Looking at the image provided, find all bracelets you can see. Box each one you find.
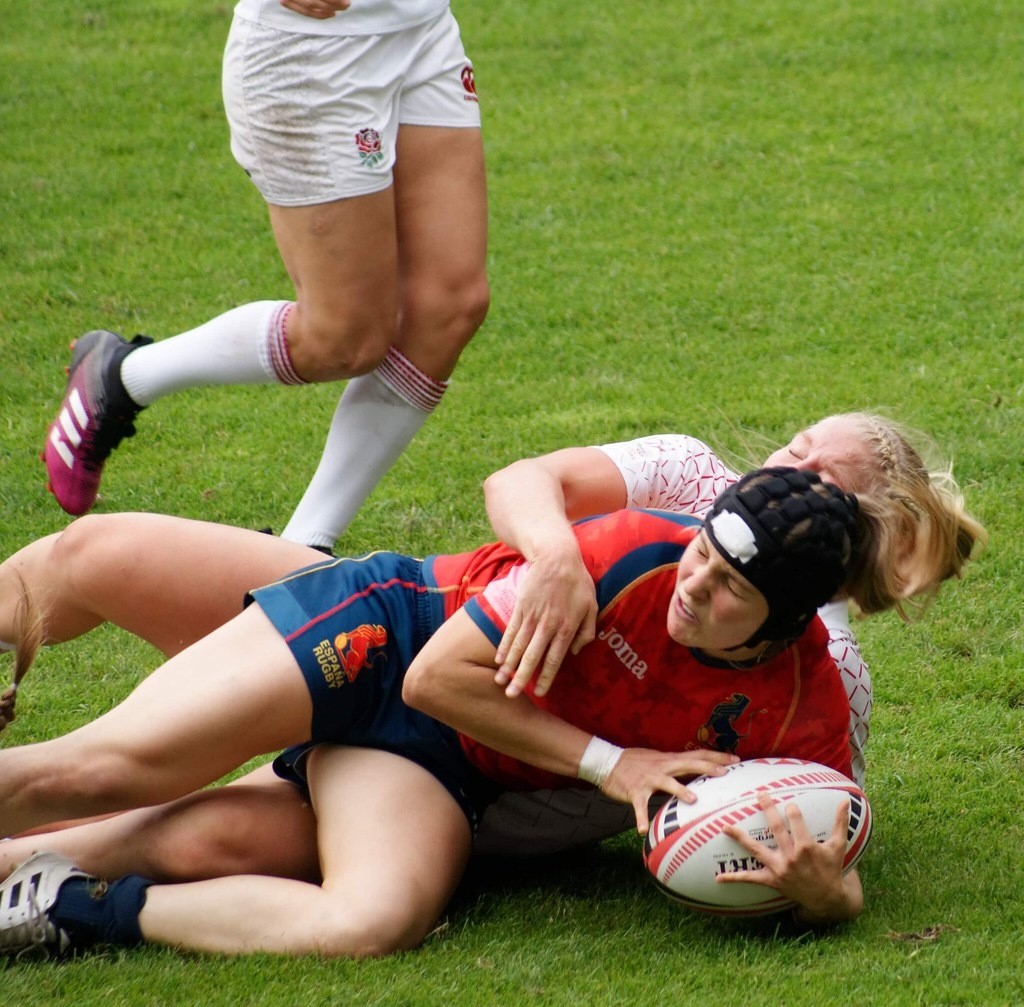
[572,734,626,788]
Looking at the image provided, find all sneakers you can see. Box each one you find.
[41,329,143,517]
[0,846,103,961]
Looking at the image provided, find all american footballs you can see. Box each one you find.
[641,756,873,915]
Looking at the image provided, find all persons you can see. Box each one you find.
[0,410,989,957]
[39,0,490,556]
